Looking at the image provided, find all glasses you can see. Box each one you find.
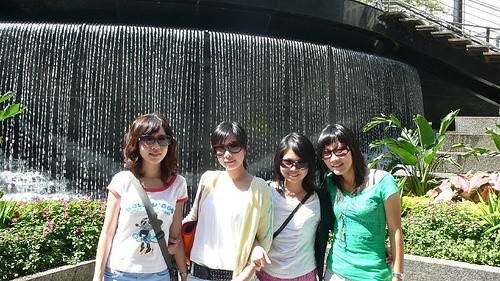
[279,158,309,170]
[138,134,172,145]
[319,145,351,160]
[213,142,243,156]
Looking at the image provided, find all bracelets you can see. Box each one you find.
[179,272,188,277]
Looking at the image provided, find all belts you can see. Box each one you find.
[189,261,233,281]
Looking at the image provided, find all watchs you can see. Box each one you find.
[392,272,405,281]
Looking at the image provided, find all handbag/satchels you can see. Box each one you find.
[181,221,198,266]
[170,254,179,281]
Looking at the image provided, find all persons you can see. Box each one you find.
[250,132,392,281]
[92,113,188,281]
[167,119,273,281]
[313,125,404,281]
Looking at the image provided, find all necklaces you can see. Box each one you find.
[285,190,305,197]
